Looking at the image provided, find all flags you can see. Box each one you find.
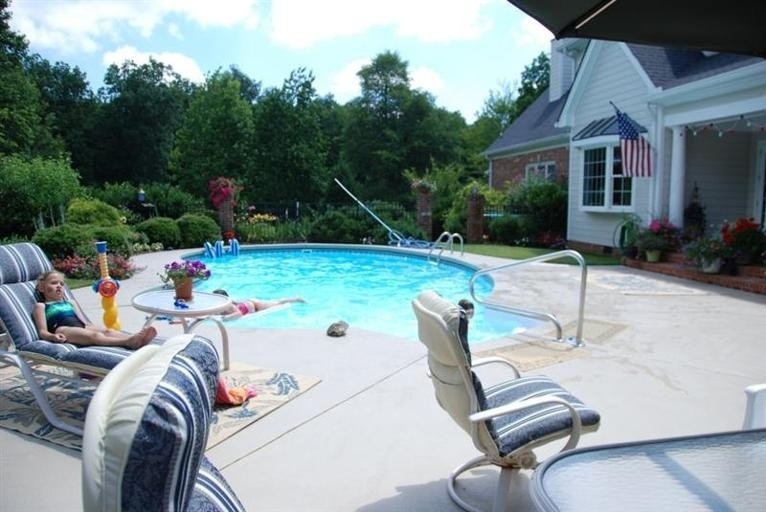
[616,109,653,178]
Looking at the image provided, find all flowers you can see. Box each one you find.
[680,234,733,269]
[156,260,211,290]
[638,218,668,251]
[720,217,766,256]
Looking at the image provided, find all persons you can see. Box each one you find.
[32,270,158,352]
[214,289,302,323]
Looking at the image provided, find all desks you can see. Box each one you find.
[131,290,232,369]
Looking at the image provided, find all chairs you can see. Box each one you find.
[0,241,137,439]
[81,334,247,511]
[412,289,600,512]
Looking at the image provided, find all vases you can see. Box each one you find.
[734,249,751,264]
[702,257,722,274]
[174,277,192,298]
[646,250,662,262]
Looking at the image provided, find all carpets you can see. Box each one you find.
[0,347,322,453]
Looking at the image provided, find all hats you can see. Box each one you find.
[327,320,349,337]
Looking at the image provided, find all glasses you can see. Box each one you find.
[174,302,190,308]
